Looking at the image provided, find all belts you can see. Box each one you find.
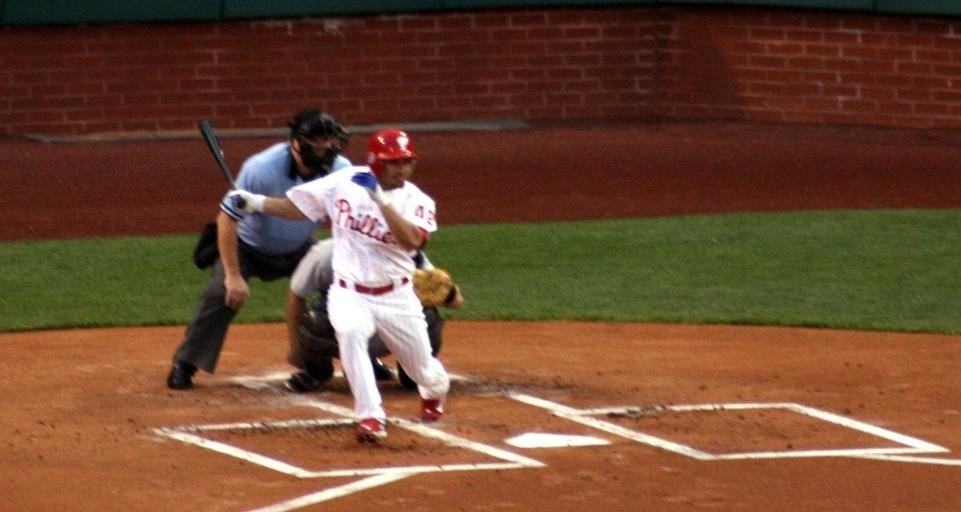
[339,278,410,296]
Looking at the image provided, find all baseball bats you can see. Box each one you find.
[198,119,246,210]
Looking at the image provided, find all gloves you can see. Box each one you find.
[351,173,388,207]
[230,188,265,212]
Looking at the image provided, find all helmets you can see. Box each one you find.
[287,110,351,177]
[367,127,419,167]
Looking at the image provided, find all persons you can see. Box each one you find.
[162,102,354,392]
[279,233,464,394]
[224,127,452,446]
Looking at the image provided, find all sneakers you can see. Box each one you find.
[354,417,388,443]
[167,360,199,390]
[370,356,419,389]
[285,366,332,393]
[419,397,445,420]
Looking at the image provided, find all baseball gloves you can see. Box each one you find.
[413,268,455,307]
